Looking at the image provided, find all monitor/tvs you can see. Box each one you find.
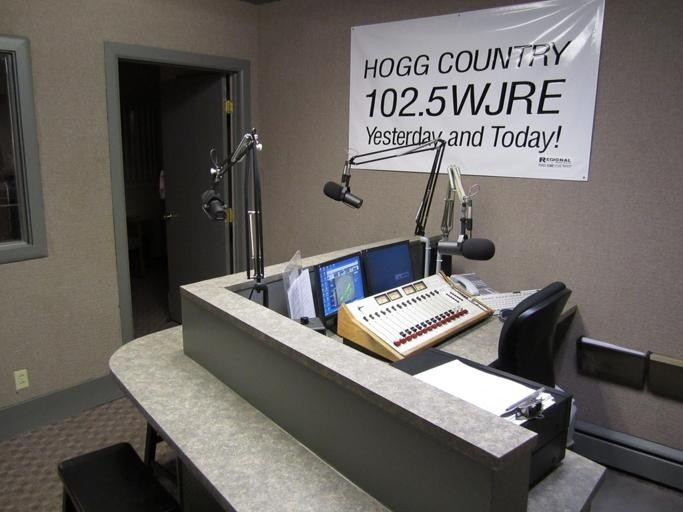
[314,251,369,324]
[362,240,415,297]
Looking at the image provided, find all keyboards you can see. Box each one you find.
[476,288,540,316]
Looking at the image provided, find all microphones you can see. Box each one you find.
[323,181,362,209]
[437,238,496,260]
[200,188,227,223]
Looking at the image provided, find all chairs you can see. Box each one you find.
[498,281,572,389]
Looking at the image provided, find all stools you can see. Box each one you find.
[58,441,183,512]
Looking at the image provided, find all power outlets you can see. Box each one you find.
[12,369,29,392]
